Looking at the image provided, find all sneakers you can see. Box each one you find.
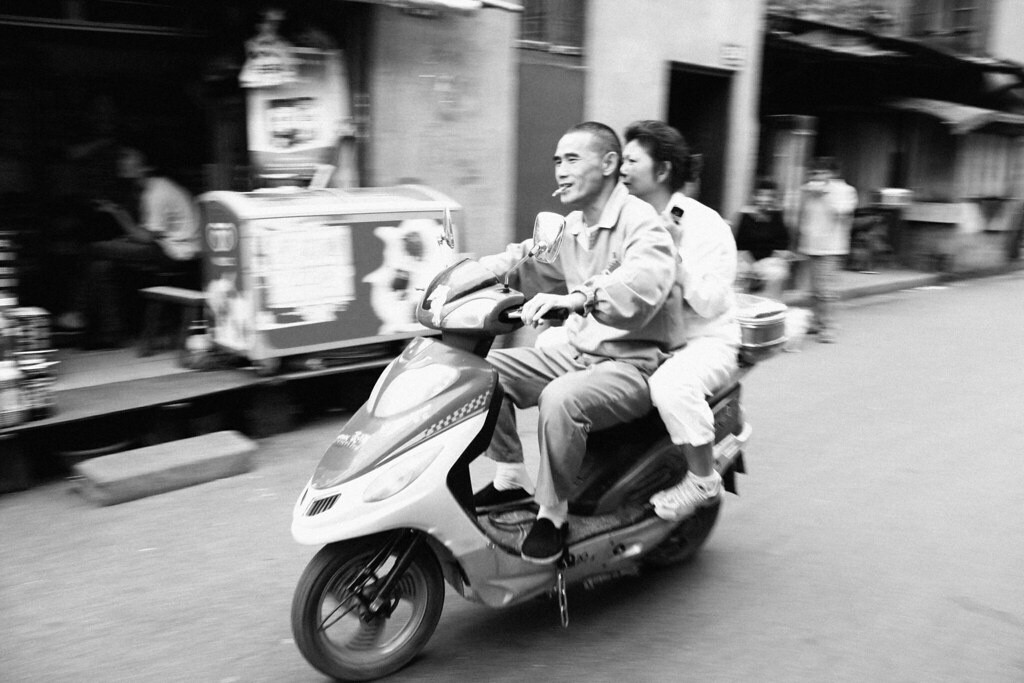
[652,472,721,521]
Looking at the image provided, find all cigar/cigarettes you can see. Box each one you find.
[551,186,567,197]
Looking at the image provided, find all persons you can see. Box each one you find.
[60,143,203,351]
[798,156,857,344]
[473,122,743,565]
[734,180,790,302]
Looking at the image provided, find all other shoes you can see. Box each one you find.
[62,312,84,328]
[820,328,835,342]
[474,482,529,513]
[521,517,570,564]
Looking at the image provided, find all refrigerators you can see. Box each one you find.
[196,185,461,360]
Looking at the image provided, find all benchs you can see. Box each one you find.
[138,285,208,357]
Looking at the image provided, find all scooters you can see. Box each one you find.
[289,205,792,683]
